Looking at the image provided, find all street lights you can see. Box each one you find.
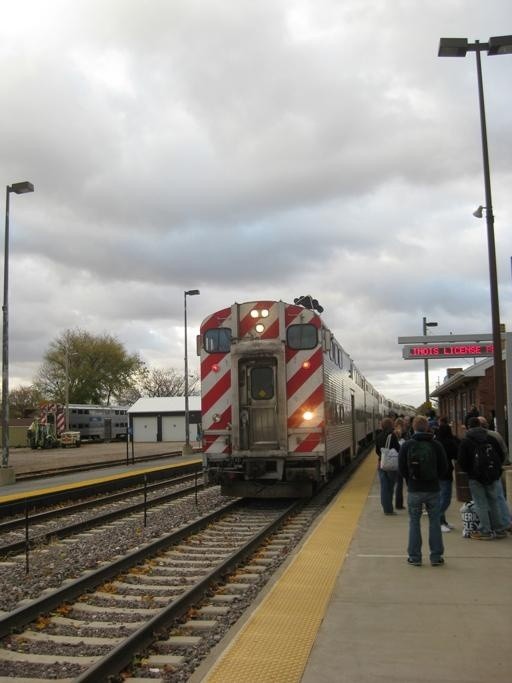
[421,317,438,402]
[437,35,512,501]
[182,290,200,456]
[0,181,35,486]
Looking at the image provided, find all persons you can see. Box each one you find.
[397,413,447,565]
[374,406,512,541]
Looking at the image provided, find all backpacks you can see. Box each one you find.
[408,439,439,482]
[463,437,503,482]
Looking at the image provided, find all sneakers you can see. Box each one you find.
[384,510,394,515]
[395,504,407,510]
[440,523,457,533]
[469,529,507,539]
[430,557,445,565]
[407,557,421,566]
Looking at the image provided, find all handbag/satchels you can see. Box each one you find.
[380,433,400,472]
[460,499,496,540]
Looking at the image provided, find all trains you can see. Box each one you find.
[197,295,416,498]
[39,403,130,439]
